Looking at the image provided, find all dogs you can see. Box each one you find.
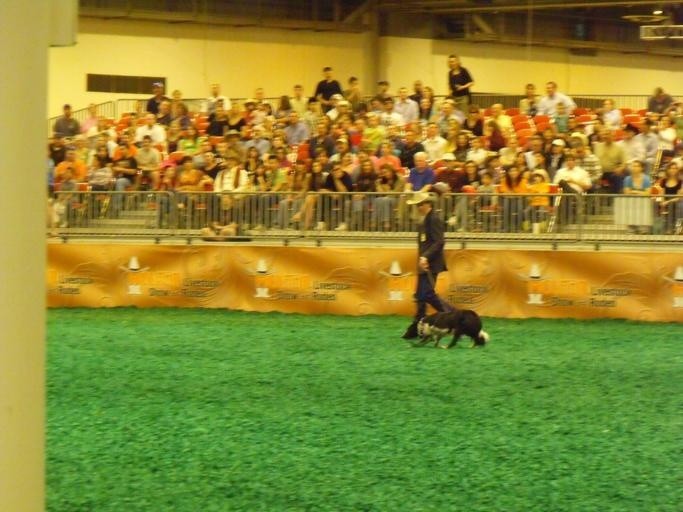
[416,309,490,349]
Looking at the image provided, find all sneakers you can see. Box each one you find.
[402,321,421,339]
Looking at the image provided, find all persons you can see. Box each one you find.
[316,66,343,102]
[48,77,683,238]
[400,191,454,338]
[446,52,474,111]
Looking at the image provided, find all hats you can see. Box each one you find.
[405,191,439,207]
